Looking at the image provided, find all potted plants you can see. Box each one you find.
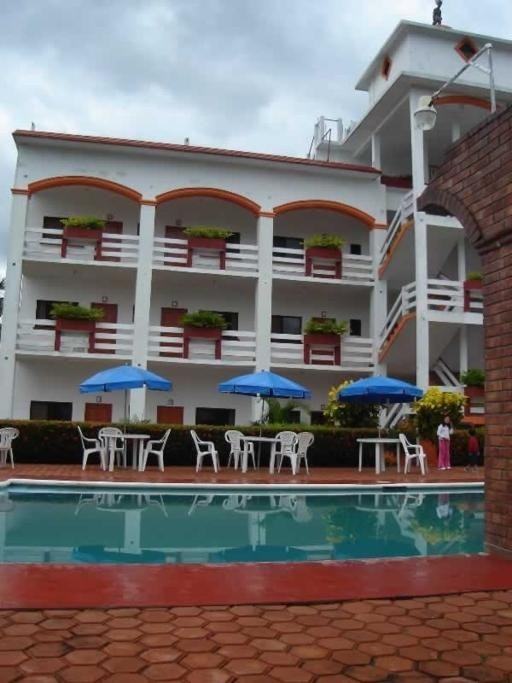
[177,306,233,338]
[460,366,486,398]
[461,267,484,290]
[303,314,350,345]
[298,230,349,259]
[48,300,107,331]
[181,224,238,251]
[59,214,110,241]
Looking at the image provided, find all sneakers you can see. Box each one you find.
[439,466,452,471]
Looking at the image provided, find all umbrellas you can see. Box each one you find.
[216,365,312,470]
[204,493,311,563]
[325,496,421,557]
[70,504,168,566]
[78,359,173,469]
[333,371,425,442]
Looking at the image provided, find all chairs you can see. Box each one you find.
[1,425,21,470]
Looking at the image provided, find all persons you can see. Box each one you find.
[434,414,455,469]
[432,0,443,25]
[436,493,453,527]
[464,427,481,472]
